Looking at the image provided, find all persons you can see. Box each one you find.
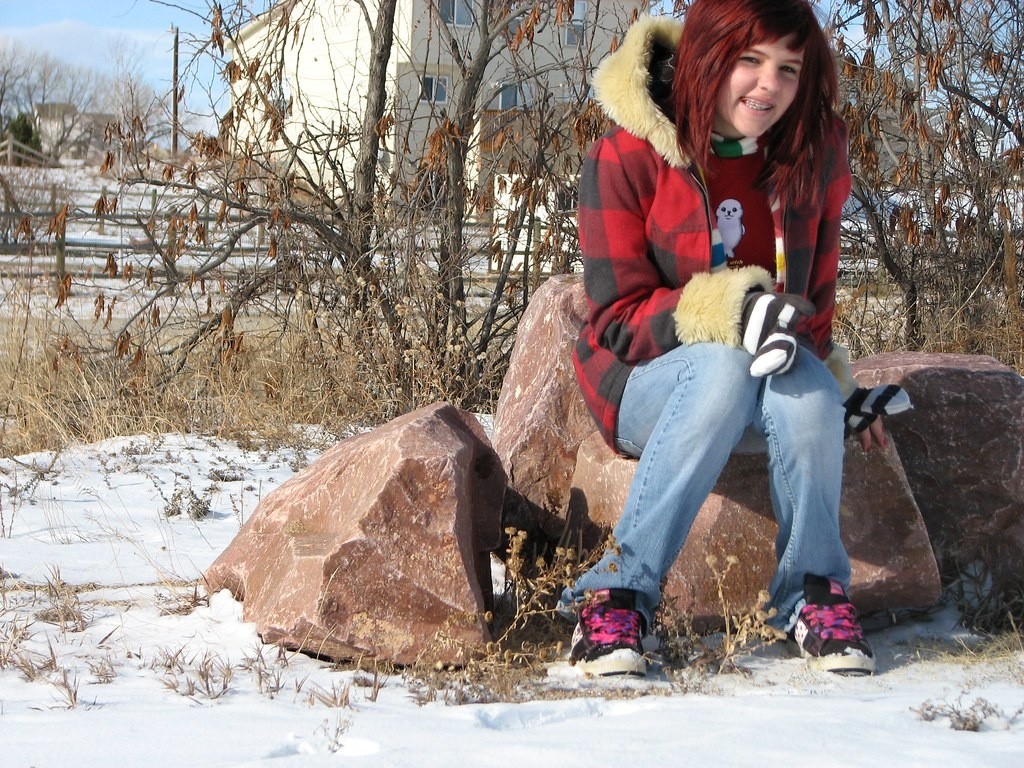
[567,0,890,678]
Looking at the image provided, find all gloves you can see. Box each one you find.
[739,290,817,378]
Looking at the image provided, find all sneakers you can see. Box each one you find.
[564,588,647,677]
[785,571,879,672]
